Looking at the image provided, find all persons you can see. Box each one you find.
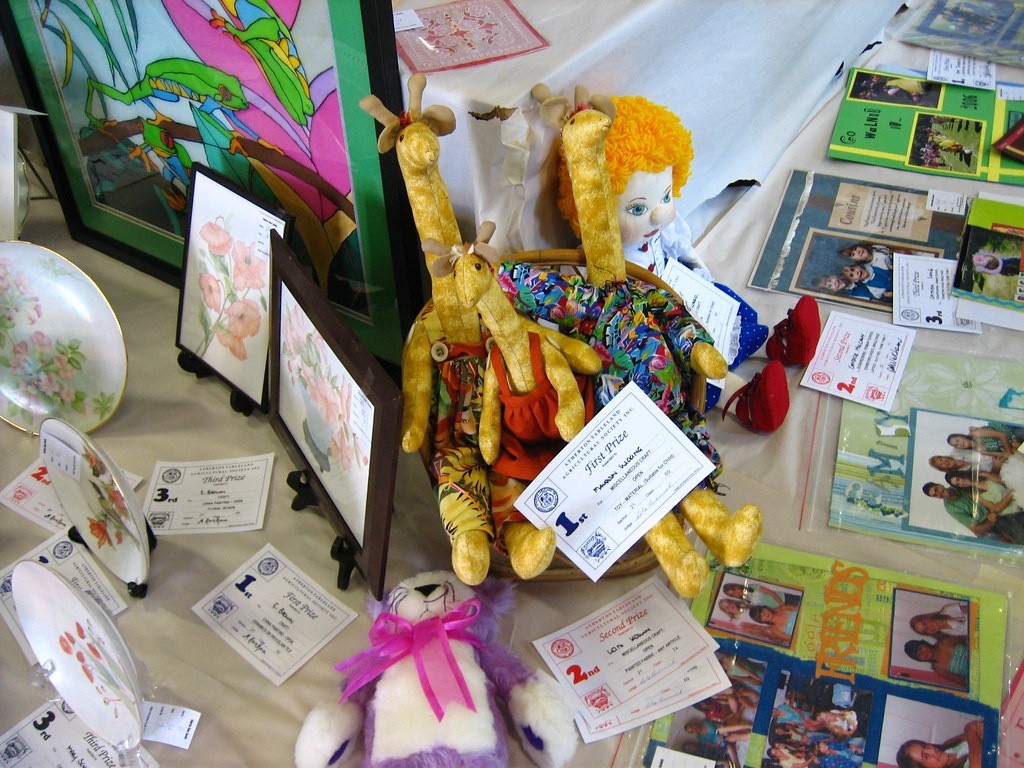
[810,0,1024,306]
[911,416,1024,547]
[658,558,985,768]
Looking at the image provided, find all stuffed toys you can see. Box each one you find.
[292,569,578,768]
[359,71,823,599]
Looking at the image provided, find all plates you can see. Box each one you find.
[11,561,144,749]
[0,241,128,437]
[39,417,150,586]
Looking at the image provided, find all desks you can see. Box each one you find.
[395,0,919,253]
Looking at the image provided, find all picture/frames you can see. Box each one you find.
[175,162,297,415]
[0,0,425,390]
[267,229,406,602]
